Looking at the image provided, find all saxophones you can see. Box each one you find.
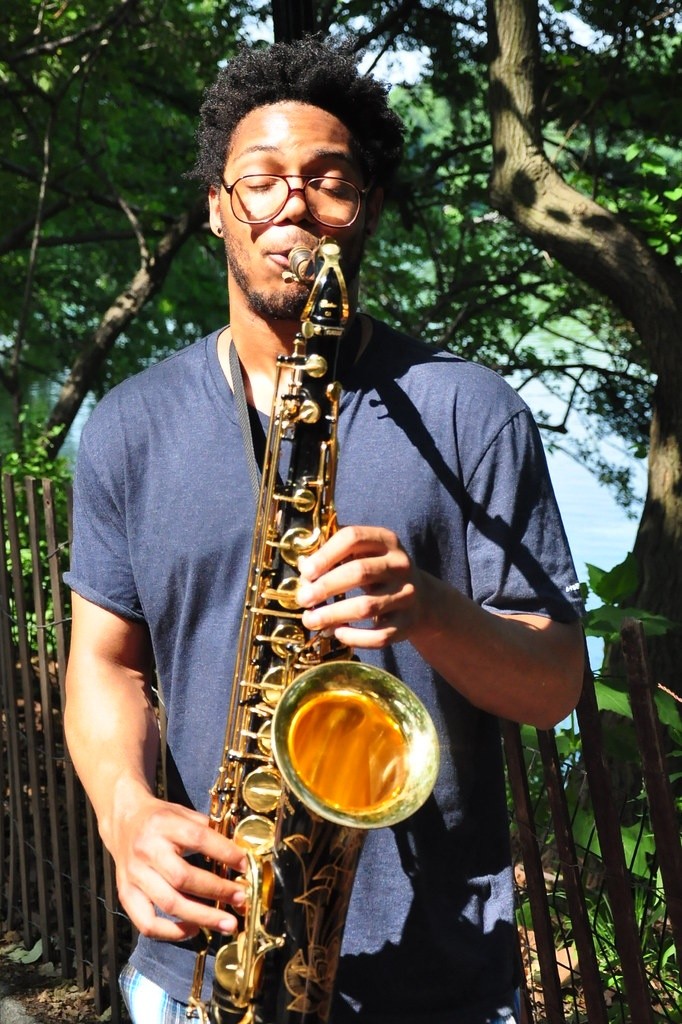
[184,235,443,1024]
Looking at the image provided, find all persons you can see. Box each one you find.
[62,29,588,1024]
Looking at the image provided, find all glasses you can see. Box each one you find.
[220,171,376,229]
